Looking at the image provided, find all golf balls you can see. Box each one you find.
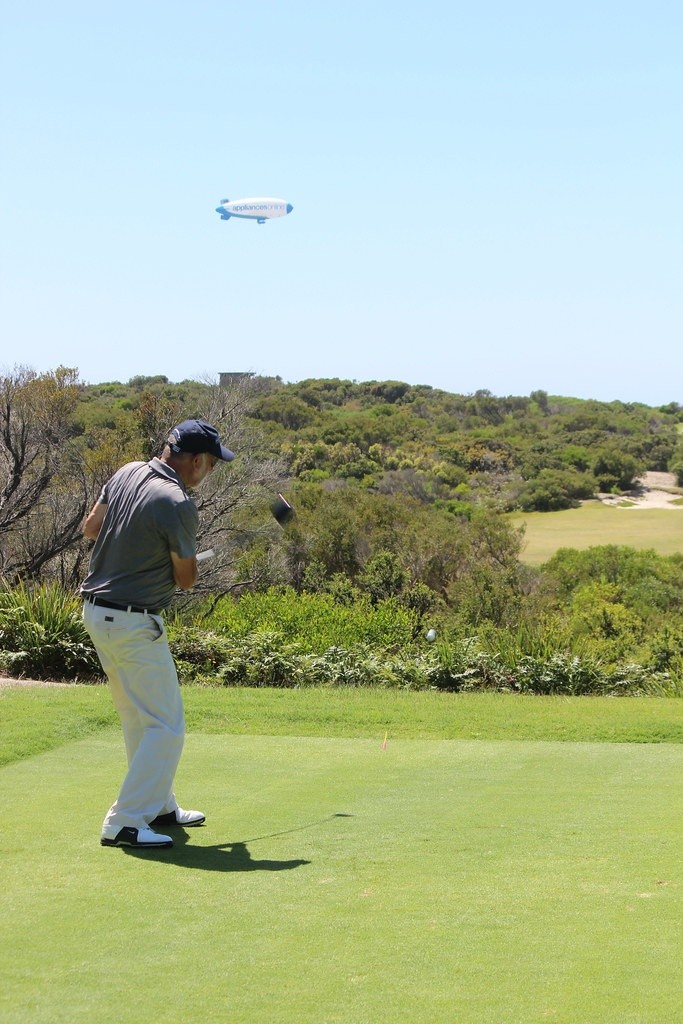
[425,629,436,642]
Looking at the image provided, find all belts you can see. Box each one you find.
[84,594,161,615]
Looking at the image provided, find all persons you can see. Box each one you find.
[80,419,234,849]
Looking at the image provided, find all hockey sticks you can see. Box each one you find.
[195,496,295,562]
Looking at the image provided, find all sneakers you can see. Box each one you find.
[148,807,205,826]
[100,826,173,847]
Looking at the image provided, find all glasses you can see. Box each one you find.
[192,452,218,467]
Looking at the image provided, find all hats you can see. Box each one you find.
[164,420,234,462]
